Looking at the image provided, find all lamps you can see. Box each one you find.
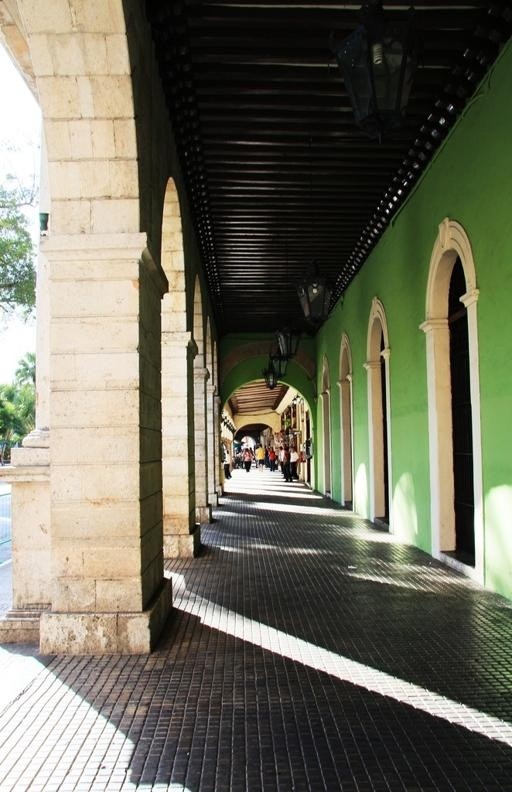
[326,1,426,141]
[263,93,335,390]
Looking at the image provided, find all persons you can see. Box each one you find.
[222,442,300,482]
